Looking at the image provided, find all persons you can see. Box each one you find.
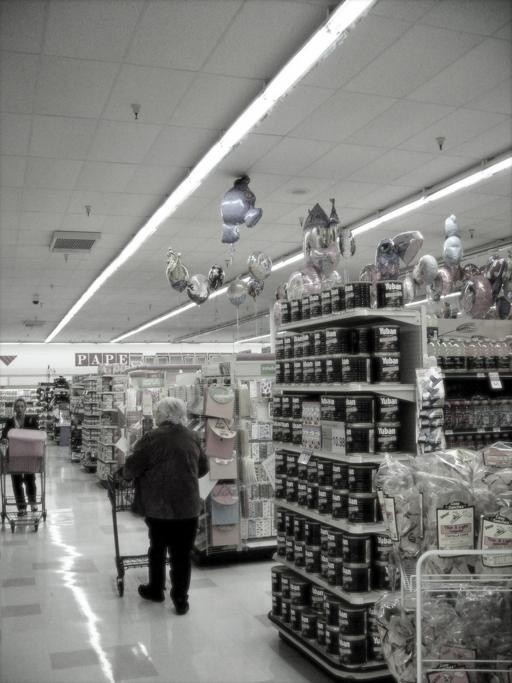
[119,396,210,615]
[0,398,39,517]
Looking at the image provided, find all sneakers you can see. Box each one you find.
[137,583,166,602]
[17,508,28,516]
[31,504,39,512]
[169,586,190,616]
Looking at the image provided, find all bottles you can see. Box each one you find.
[80,380,101,454]
[429,336,512,451]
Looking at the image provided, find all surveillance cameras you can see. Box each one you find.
[33,300,39,305]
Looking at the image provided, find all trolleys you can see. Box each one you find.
[105,470,173,599]
[0,436,49,535]
[389,539,511,683]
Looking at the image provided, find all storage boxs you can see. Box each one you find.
[8,428,46,457]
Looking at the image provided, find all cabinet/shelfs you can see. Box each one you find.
[263,305,421,680]
[1,382,41,423]
[427,354,512,451]
[43,366,280,560]
